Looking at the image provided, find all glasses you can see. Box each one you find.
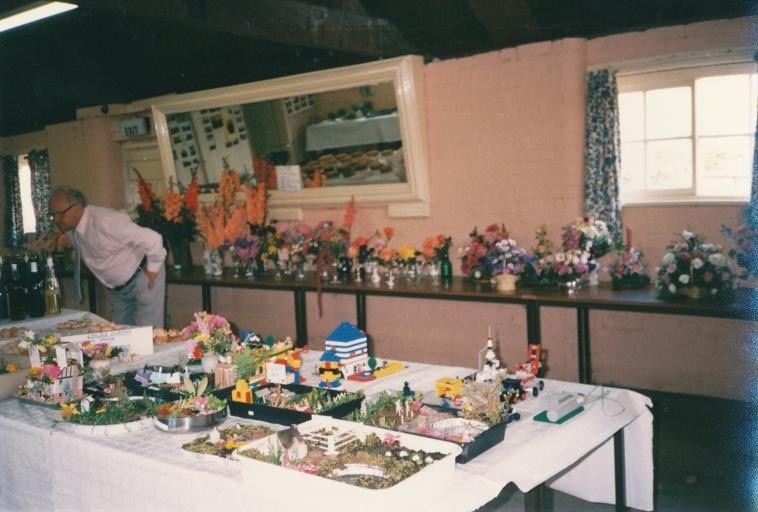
[46,204,76,221]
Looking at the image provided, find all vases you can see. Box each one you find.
[202,251,453,287]
[489,273,519,291]
[162,230,192,280]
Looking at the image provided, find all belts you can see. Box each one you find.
[105,256,147,292]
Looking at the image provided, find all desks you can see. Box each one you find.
[2,308,113,341]
[0,340,628,512]
[306,110,401,154]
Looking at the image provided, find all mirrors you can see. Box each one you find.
[150,54,429,207]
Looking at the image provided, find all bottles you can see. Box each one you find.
[317,245,452,284]
[0,253,62,321]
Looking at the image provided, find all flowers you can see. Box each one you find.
[459,215,646,279]
[194,163,448,263]
[656,228,738,299]
[133,162,207,245]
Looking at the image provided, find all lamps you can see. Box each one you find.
[0,0,79,34]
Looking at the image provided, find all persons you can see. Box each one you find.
[49,186,169,331]
